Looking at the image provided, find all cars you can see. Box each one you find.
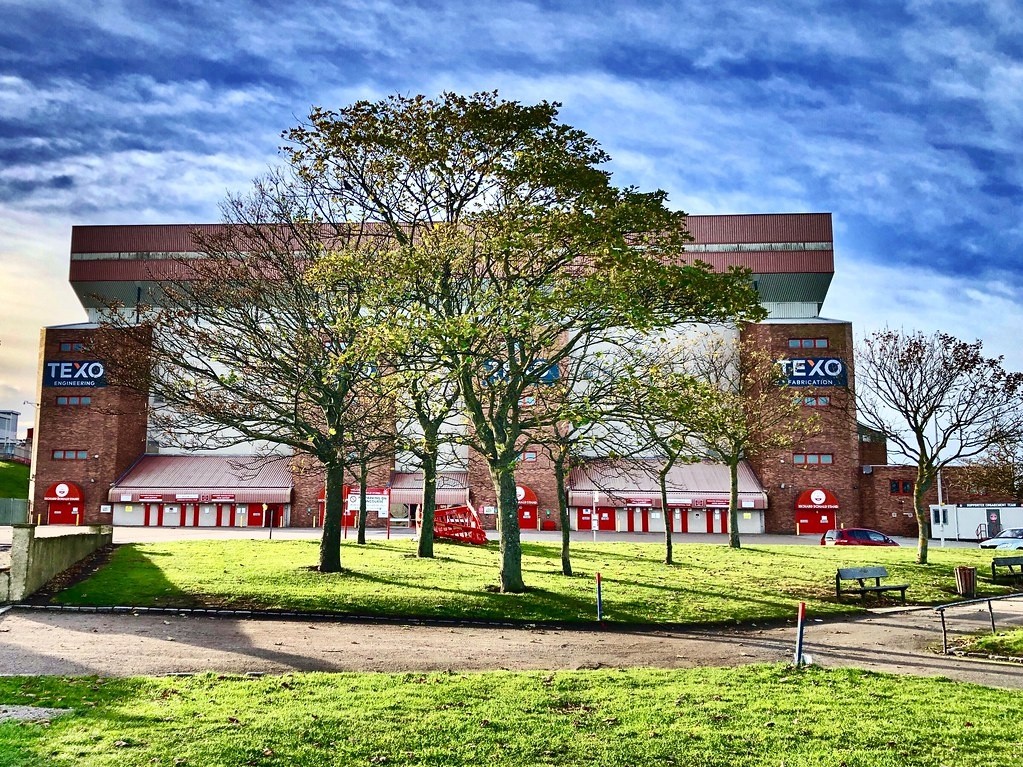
[995,539,1023,550]
[820,528,900,546]
[978,527,1023,550]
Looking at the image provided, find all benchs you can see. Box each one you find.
[991,556,1023,581]
[836,567,908,604]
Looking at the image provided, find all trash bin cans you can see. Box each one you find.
[955,567,976,597]
